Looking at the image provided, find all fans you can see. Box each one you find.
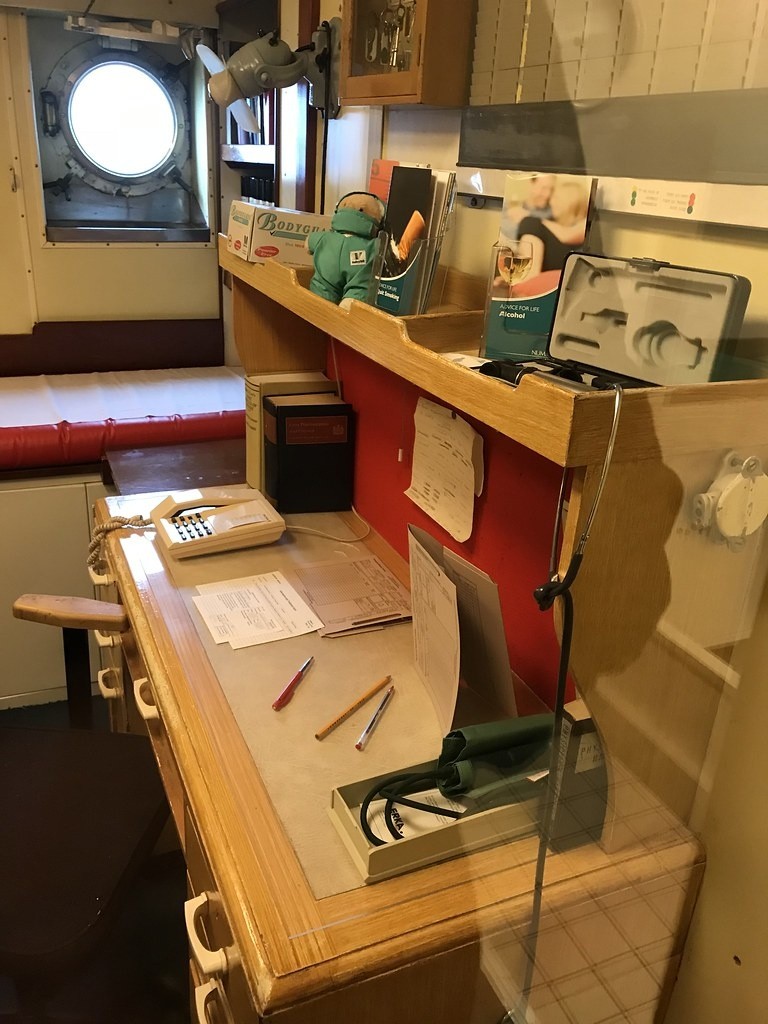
[195,22,332,134]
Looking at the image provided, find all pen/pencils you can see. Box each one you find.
[272,655,315,711]
[315,674,395,750]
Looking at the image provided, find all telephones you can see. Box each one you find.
[148,487,288,559]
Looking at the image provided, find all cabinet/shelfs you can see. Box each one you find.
[0,476,109,710]
[337,0,473,108]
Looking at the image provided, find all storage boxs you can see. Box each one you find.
[226,200,333,267]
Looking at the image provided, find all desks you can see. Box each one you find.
[89,482,705,1024]
